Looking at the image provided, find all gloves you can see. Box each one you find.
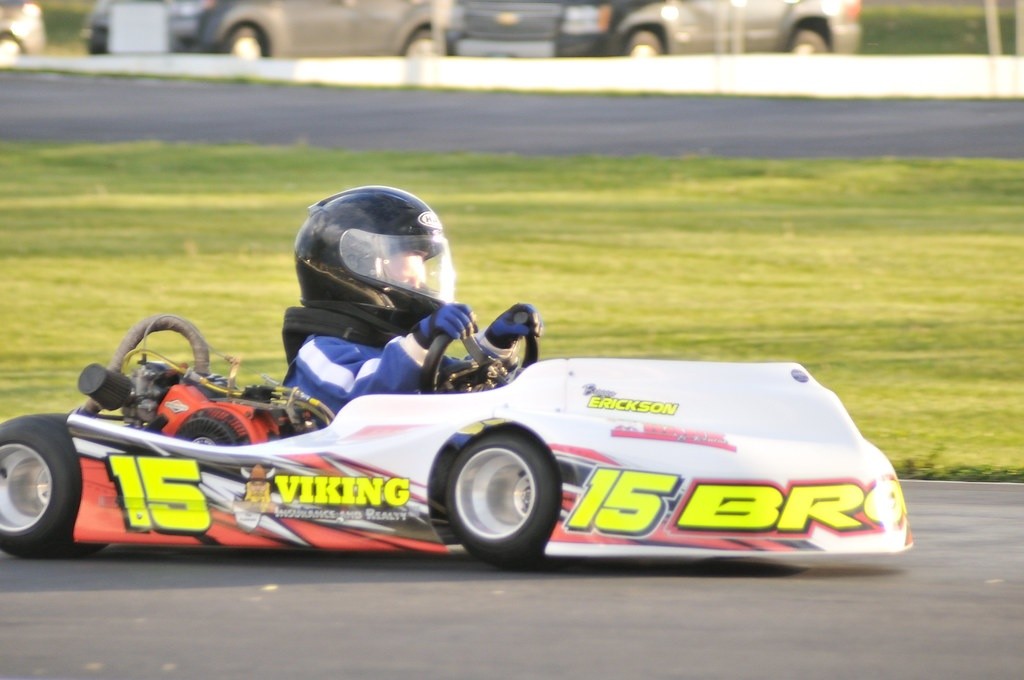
[414,302,478,349]
[485,303,542,349]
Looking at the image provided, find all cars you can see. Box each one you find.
[430,1,861,58]
[85,0,430,59]
[0,0,44,62]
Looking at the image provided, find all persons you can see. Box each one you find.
[281,183,541,416]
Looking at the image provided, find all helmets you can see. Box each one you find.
[295,185,455,332]
